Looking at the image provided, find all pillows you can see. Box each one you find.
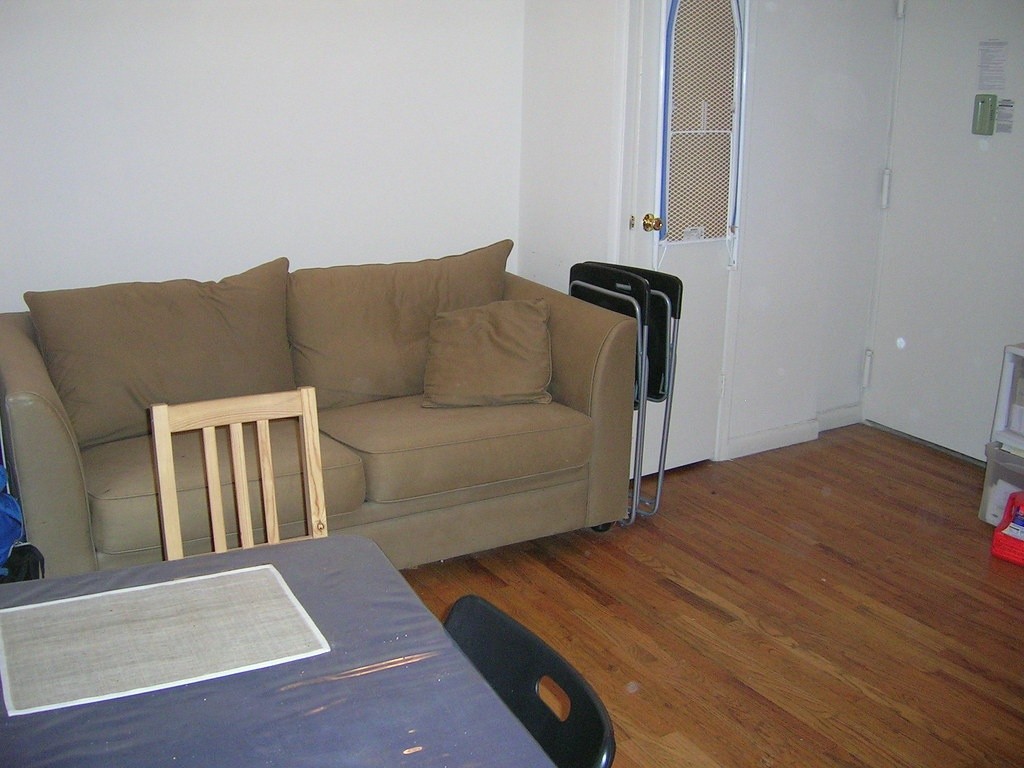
[22,258,296,448]
[288,239,514,407]
[420,295,553,408]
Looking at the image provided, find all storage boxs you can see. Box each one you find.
[979,342,1024,527]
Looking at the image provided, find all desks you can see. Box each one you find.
[0,534,557,767]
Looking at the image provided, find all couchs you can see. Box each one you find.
[0,270,638,578]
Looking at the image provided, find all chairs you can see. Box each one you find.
[445,595,616,768]
[150,386,328,561]
[568,259,683,536]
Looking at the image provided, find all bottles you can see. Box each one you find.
[991,490,1024,566]
